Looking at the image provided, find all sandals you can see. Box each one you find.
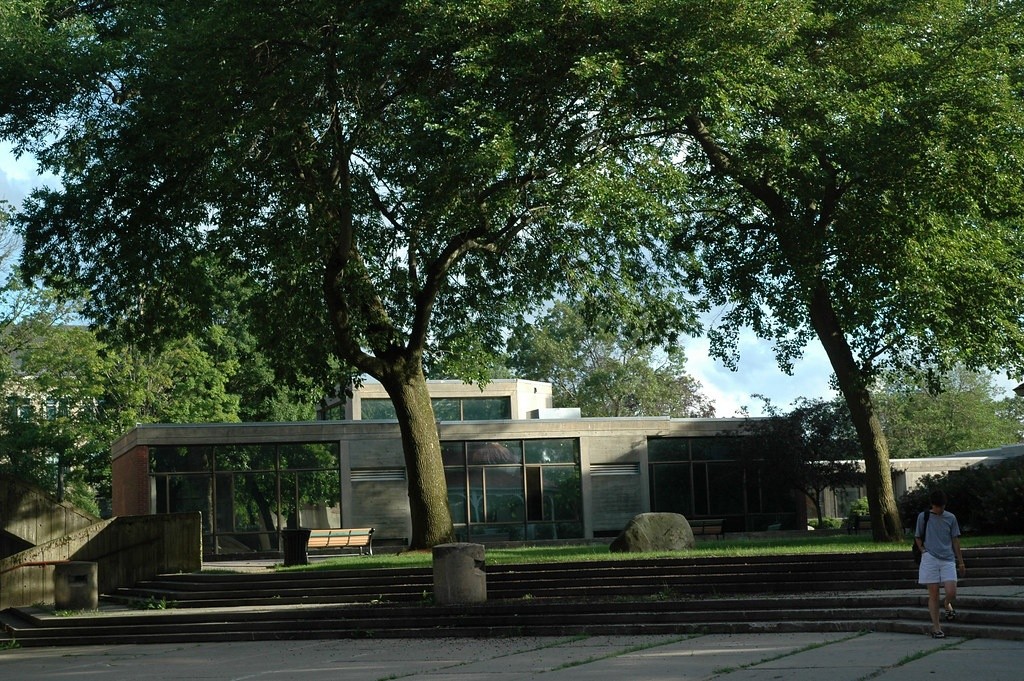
[931,630,945,639]
[945,609,955,622]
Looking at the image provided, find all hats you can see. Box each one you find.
[929,489,947,506]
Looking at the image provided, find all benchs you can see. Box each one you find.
[305,527,376,557]
[688,519,727,541]
[848,515,872,536]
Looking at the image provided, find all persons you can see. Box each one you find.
[915,491,966,638]
[847,512,859,535]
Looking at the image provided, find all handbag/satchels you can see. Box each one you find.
[912,539,923,565]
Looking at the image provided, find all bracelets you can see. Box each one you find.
[960,564,964,566]
